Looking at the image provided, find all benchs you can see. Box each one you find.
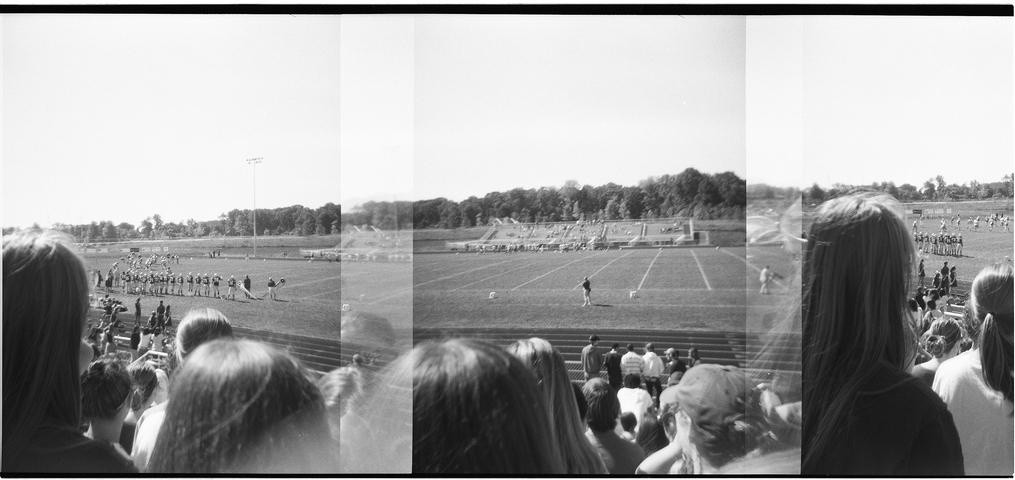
[474,218,693,248]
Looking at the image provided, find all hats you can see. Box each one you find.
[659,361,764,423]
[662,346,679,357]
[666,370,685,387]
[589,334,601,342]
